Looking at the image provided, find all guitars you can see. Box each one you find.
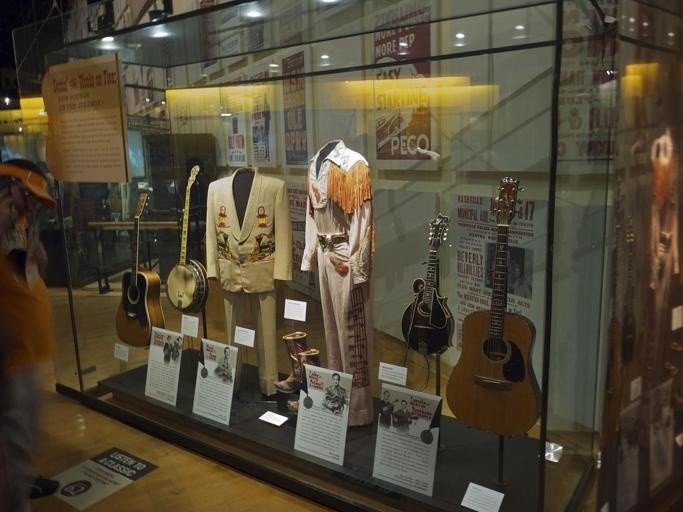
[401,213,454,355]
[116,193,165,346]
[446,177,542,434]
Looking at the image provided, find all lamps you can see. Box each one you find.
[97,15,113,43]
[148,10,170,39]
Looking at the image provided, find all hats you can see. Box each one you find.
[0,164,58,210]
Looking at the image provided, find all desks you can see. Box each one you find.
[88,220,179,295]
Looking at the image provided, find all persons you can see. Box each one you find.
[0,158,59,512]
[171,336,182,362]
[393,400,412,432]
[216,347,232,375]
[261,92,271,163]
[321,373,345,414]
[375,391,393,430]
[162,335,172,363]
